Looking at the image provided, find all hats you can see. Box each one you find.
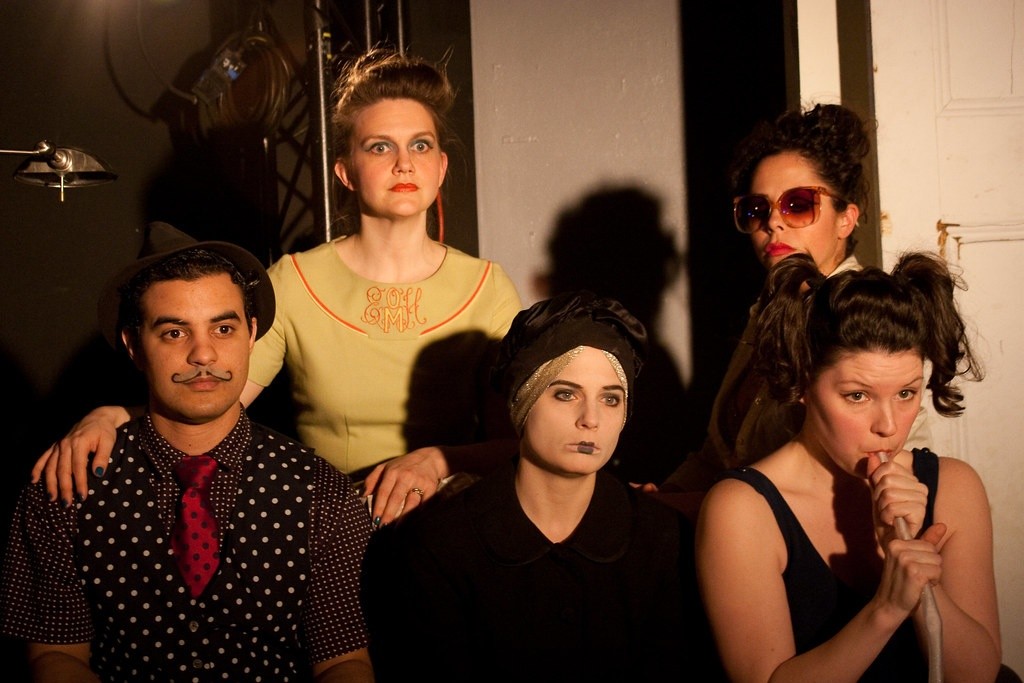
[97,220,276,351]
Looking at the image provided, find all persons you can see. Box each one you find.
[363,295,720,683]
[31,45,523,526]
[705,105,874,475]
[2,220,376,683]
[698,252,1003,683]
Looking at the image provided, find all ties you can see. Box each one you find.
[170,456,221,594]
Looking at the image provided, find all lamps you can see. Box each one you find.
[0,140,118,203]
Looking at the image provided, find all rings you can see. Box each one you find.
[408,487,423,501]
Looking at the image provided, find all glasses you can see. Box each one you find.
[732,186,844,230]
